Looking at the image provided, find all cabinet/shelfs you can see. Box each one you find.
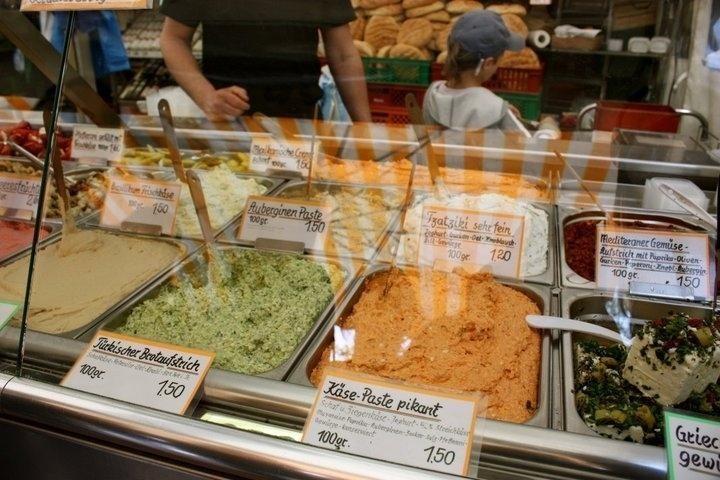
[543,1,676,113]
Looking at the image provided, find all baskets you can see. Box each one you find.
[360,57,431,124]
[432,63,544,122]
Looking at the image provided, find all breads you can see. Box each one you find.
[317,0,541,91]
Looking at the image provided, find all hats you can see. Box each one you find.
[448,9,526,63]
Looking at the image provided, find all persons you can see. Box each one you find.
[421,10,563,141]
[157,0,375,125]
